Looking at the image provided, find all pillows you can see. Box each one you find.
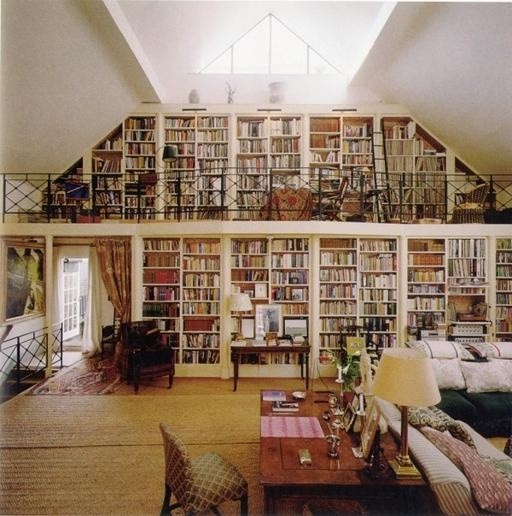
[434,357,467,390]
[395,404,512,516]
[460,359,511,396]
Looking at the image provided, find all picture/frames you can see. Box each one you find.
[340,401,356,433]
[0,240,47,327]
[358,397,382,460]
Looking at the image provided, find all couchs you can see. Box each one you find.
[372,395,511,516]
[436,352,512,438]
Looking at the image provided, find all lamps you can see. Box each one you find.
[369,346,442,480]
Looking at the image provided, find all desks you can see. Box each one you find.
[258,389,426,515]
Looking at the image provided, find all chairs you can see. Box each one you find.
[117,320,176,394]
[159,422,249,516]
[101,325,115,355]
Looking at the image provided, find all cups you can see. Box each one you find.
[328,394,337,407]
[326,435,341,457]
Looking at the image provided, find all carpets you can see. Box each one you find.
[24,350,123,397]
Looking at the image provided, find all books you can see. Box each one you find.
[309,123,373,192]
[91,123,122,219]
[385,121,446,219]
[43,157,83,219]
[495,238,512,342]
[144,240,220,363]
[165,117,227,219]
[230,240,308,363]
[237,118,300,220]
[318,238,398,364]
[408,238,488,341]
[125,118,156,220]
